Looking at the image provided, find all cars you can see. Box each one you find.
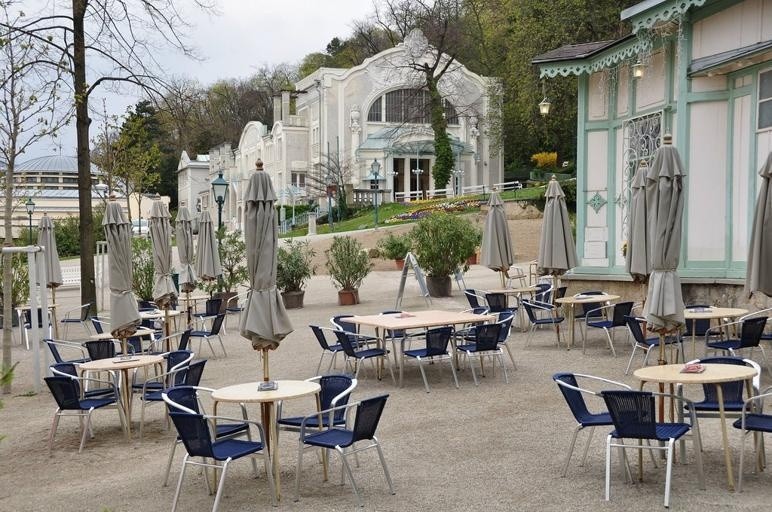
[561,161,568,169]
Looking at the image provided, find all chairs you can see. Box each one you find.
[675,354,764,468]
[552,369,657,483]
[2,293,248,453]
[293,392,396,508]
[167,410,278,512]
[600,388,707,509]
[732,383,772,493]
[304,306,515,391]
[280,374,358,480]
[464,262,772,376]
[160,381,259,486]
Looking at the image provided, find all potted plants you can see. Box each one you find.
[375,233,411,272]
[405,207,481,298]
[276,233,318,309]
[191,224,251,310]
[1,259,31,329]
[128,237,156,311]
[323,234,375,305]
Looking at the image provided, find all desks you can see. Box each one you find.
[209,380,328,493]
[631,363,766,492]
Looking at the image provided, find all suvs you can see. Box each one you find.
[130,219,152,240]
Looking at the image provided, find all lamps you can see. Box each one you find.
[631,48,649,79]
[538,76,552,114]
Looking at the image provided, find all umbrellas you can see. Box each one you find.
[32,211,64,337]
[643,134,688,460]
[102,196,143,428]
[195,206,222,300]
[147,193,179,352]
[239,157,293,458]
[478,187,514,290]
[535,174,579,342]
[623,160,651,354]
[174,202,198,331]
[743,146,771,301]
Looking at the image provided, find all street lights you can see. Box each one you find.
[450,168,464,196]
[25,198,37,246]
[384,171,398,202]
[211,170,230,268]
[412,168,424,200]
[370,157,381,228]
